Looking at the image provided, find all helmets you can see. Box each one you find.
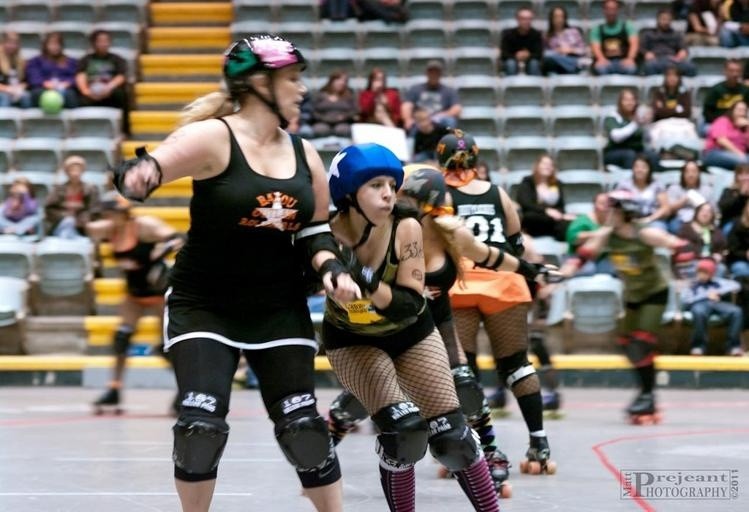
[605,186,654,220]
[329,142,404,206]
[224,35,305,99]
[100,191,131,212]
[436,128,482,170]
[398,169,446,207]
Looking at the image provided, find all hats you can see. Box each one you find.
[698,260,714,275]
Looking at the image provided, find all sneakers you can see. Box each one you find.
[692,347,705,355]
[729,346,742,355]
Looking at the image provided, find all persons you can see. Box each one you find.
[1,3,749,511]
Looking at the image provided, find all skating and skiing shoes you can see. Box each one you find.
[542,391,562,417]
[487,389,507,417]
[484,449,512,499]
[520,433,558,474]
[627,393,662,423]
[95,388,126,415]
